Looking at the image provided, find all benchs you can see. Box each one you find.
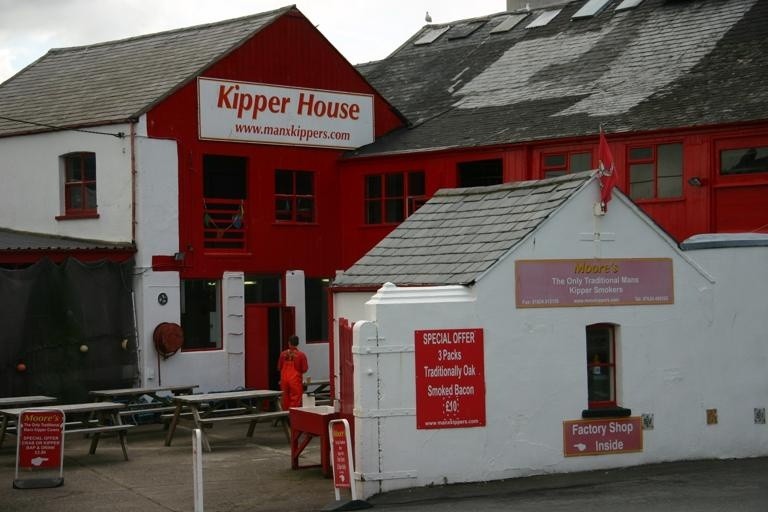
[0,408,291,462]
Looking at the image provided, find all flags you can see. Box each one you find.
[595,130,620,206]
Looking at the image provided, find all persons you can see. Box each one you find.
[275,334,310,411]
